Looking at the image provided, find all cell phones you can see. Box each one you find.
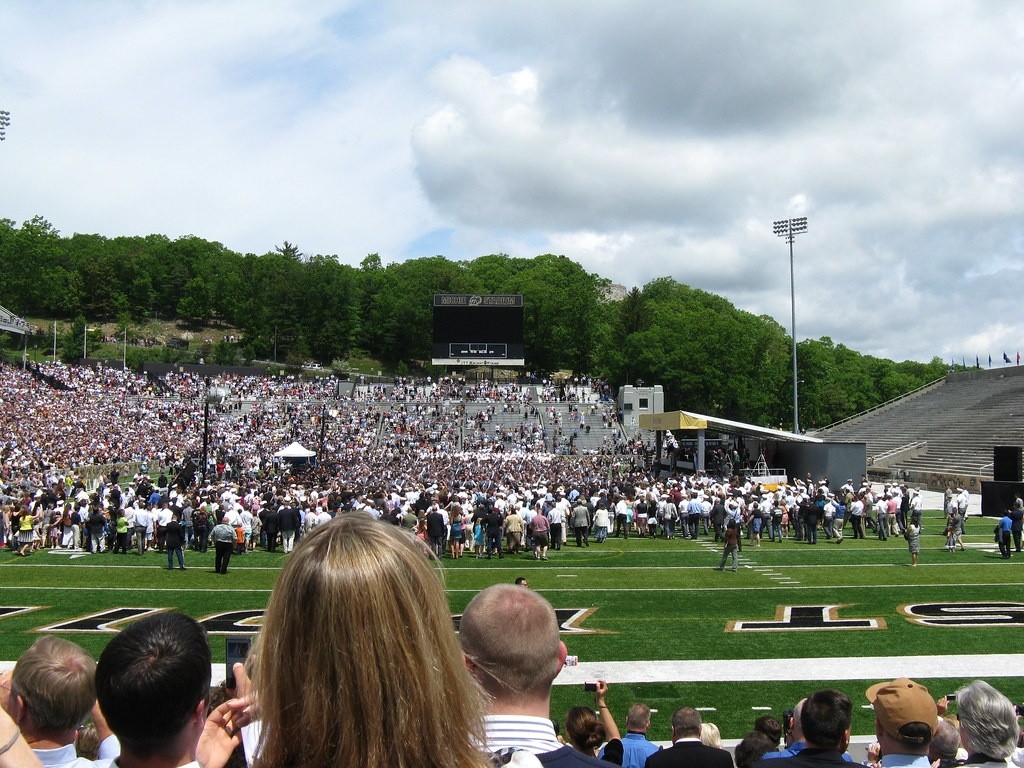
[226,638,252,689]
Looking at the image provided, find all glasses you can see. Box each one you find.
[1,680,32,707]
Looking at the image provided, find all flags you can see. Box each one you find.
[951,350,1021,368]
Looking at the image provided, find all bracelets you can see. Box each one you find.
[0,726,21,754]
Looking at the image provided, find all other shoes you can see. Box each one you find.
[180,565,187,570]
[883,537,887,541]
[872,530,877,533]
[4,533,280,556]
[718,567,724,570]
[551,532,700,550]
[778,540,782,543]
[756,545,760,547]
[426,543,532,559]
[851,537,857,539]
[806,542,811,544]
[542,555,548,560]
[748,543,753,545]
[826,535,832,539]
[770,540,775,542]
[168,565,172,568]
[813,541,816,544]
[859,537,863,539]
[836,538,843,544]
[535,551,538,558]
[731,567,736,571]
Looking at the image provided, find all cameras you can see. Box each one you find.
[585,682,601,692]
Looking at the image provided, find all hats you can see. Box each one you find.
[847,478,852,481]
[818,480,826,484]
[866,679,940,744]
[225,496,230,499]
[235,496,241,499]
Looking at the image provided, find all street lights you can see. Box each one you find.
[772,216,808,435]
[202,378,225,478]
[319,403,338,462]
[84,326,95,360]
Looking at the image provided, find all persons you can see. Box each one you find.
[248,511,498,768]
[206,333,246,345]
[1008,492,1024,553]
[208,517,237,573]
[100,333,163,346]
[0,361,657,559]
[904,517,921,566]
[656,441,924,544]
[0,579,615,768]
[164,514,187,570]
[941,484,969,553]
[716,519,738,571]
[552,679,1024,768]
[994,512,1012,560]
